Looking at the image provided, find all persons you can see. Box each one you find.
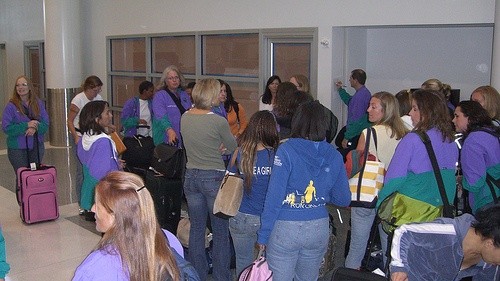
[255,101,352,281]
[227,109,280,276]
[78,100,126,211]
[121,80,155,160]
[154,66,372,152]
[390,201,500,281]
[2,76,49,206]
[180,77,238,281]
[68,75,110,220]
[338,78,500,270]
[70,171,186,281]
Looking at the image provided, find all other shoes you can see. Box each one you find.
[77,206,97,223]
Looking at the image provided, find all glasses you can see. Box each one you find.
[92,89,102,94]
[169,75,180,81]
[16,82,28,87]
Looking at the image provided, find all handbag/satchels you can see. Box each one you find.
[377,195,441,235]
[236,246,274,281]
[341,126,386,209]
[154,140,184,179]
[212,147,245,217]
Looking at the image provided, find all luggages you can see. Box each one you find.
[329,215,399,281]
[120,125,154,172]
[15,130,60,225]
[143,165,183,234]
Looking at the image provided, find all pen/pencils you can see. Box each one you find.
[336,82,346,88]
